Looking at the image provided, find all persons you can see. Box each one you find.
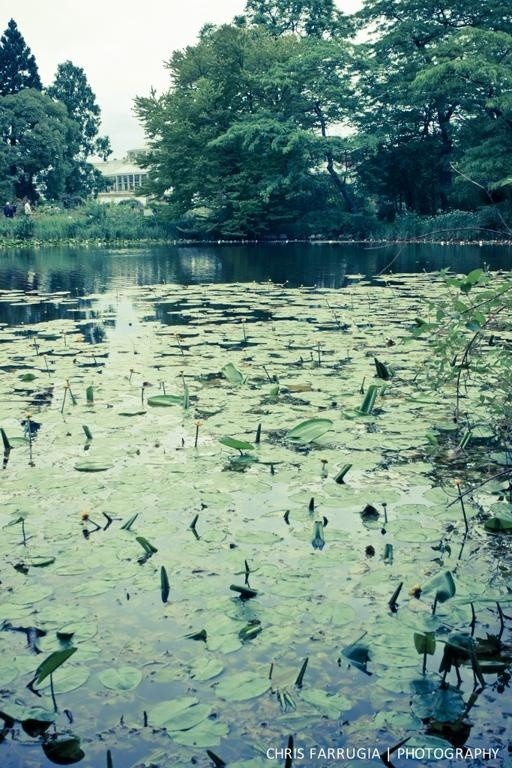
[5,201,15,217]
[24,198,32,216]
